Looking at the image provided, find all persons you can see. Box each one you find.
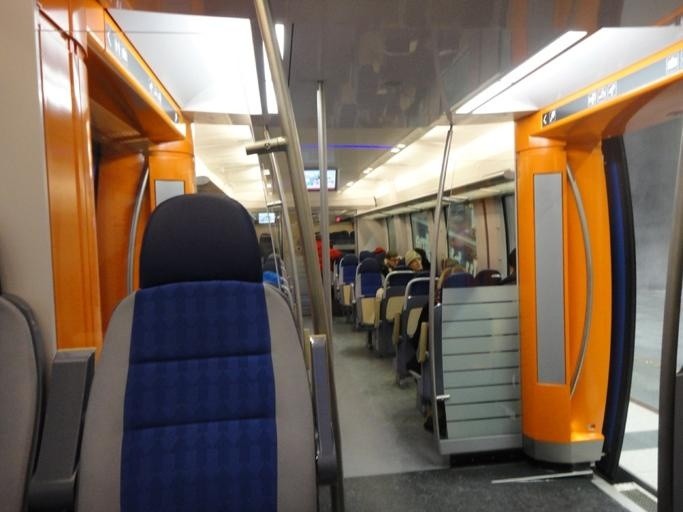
[371,245,430,276]
[258,233,274,254]
[329,241,344,259]
[408,265,468,343]
[261,253,285,293]
[497,247,516,284]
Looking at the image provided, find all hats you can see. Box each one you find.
[404,250,421,266]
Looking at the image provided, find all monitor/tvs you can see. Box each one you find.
[257,211,278,225]
[304,168,340,192]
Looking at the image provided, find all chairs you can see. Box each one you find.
[0,291,97,512]
[26,192,337,512]
[334,252,502,407]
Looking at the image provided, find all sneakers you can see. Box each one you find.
[423,416,434,431]
